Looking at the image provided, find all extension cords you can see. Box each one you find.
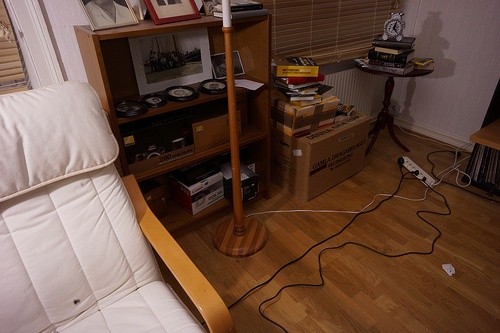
[401,156,436,187]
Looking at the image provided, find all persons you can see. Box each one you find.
[149,50,186,72]
[84,0,135,27]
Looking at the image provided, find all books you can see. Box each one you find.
[271,56,354,124]
[213,2,269,18]
[364,36,434,76]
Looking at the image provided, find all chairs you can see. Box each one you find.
[0,80,235,333]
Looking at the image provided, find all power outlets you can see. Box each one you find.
[389,103,400,115]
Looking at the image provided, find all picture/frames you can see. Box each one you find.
[128,27,213,95]
[144,0,202,25]
[78,1,139,31]
[211,50,245,79]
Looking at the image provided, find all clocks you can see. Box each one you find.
[382,12,405,41]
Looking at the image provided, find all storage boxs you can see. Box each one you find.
[193,105,241,153]
[272,92,373,202]
[221,161,258,205]
[129,143,194,174]
[168,161,224,215]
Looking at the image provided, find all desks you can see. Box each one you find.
[470,119,500,151]
[354,56,434,157]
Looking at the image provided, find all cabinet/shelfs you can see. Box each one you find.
[73,9,272,228]
